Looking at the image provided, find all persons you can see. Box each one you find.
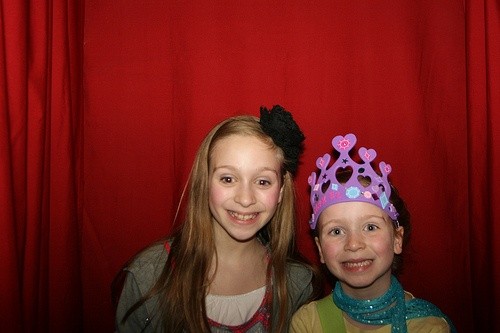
[110,103,321,332]
[288,136,458,332]
